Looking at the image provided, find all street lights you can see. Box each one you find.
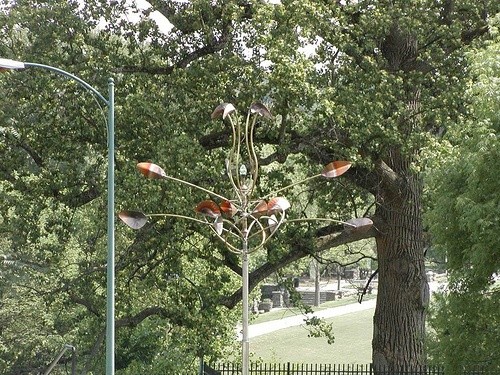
[0,55,116,375]
[116,102,375,375]
